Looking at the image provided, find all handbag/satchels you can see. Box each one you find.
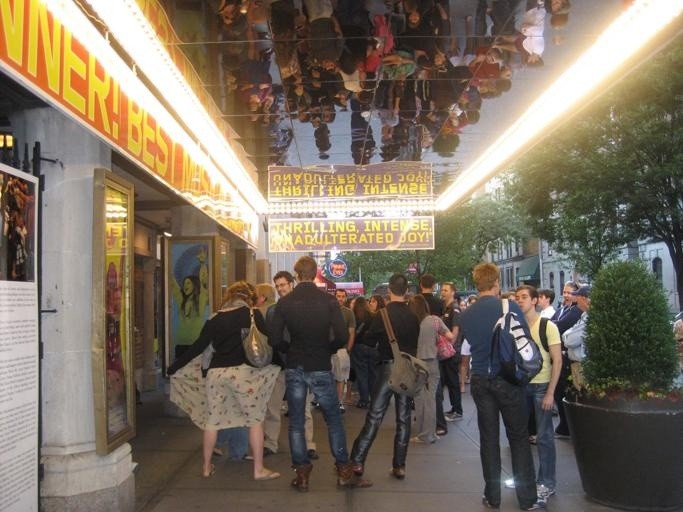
[386,350,430,399]
[239,306,274,368]
[430,315,457,362]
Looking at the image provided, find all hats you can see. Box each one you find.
[565,284,595,299]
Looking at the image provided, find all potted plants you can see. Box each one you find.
[561,258,683,512]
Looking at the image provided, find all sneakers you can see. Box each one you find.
[332,463,365,478]
[409,436,428,445]
[552,428,571,441]
[241,453,254,463]
[434,425,446,436]
[211,445,222,456]
[527,431,538,444]
[260,446,274,457]
[390,464,407,480]
[523,481,556,512]
[442,408,463,424]
[481,494,499,510]
[337,399,373,413]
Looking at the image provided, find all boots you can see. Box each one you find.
[288,462,313,494]
[332,461,374,490]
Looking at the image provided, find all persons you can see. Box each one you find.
[162,255,596,510]
[208,0,572,164]
[666,311,683,389]
[169,245,208,359]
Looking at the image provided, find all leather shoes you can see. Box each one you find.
[307,447,320,461]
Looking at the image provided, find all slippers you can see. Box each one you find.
[202,462,215,478]
[252,469,281,483]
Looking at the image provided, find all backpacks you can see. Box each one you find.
[485,298,544,389]
